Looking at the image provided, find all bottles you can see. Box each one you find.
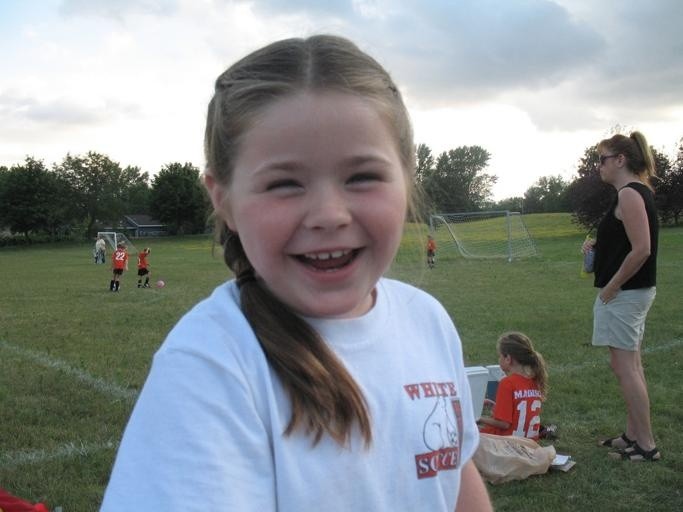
[580,243,594,279]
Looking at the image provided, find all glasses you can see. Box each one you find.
[599,156,617,164]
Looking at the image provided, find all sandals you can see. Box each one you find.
[600,434,636,447]
[608,443,660,462]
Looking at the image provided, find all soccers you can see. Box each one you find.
[156,281,164,287]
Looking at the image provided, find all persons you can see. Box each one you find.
[92,233,151,293]
[426,236,435,269]
[476,335,547,442]
[97,36,496,512]
[580,133,661,464]
